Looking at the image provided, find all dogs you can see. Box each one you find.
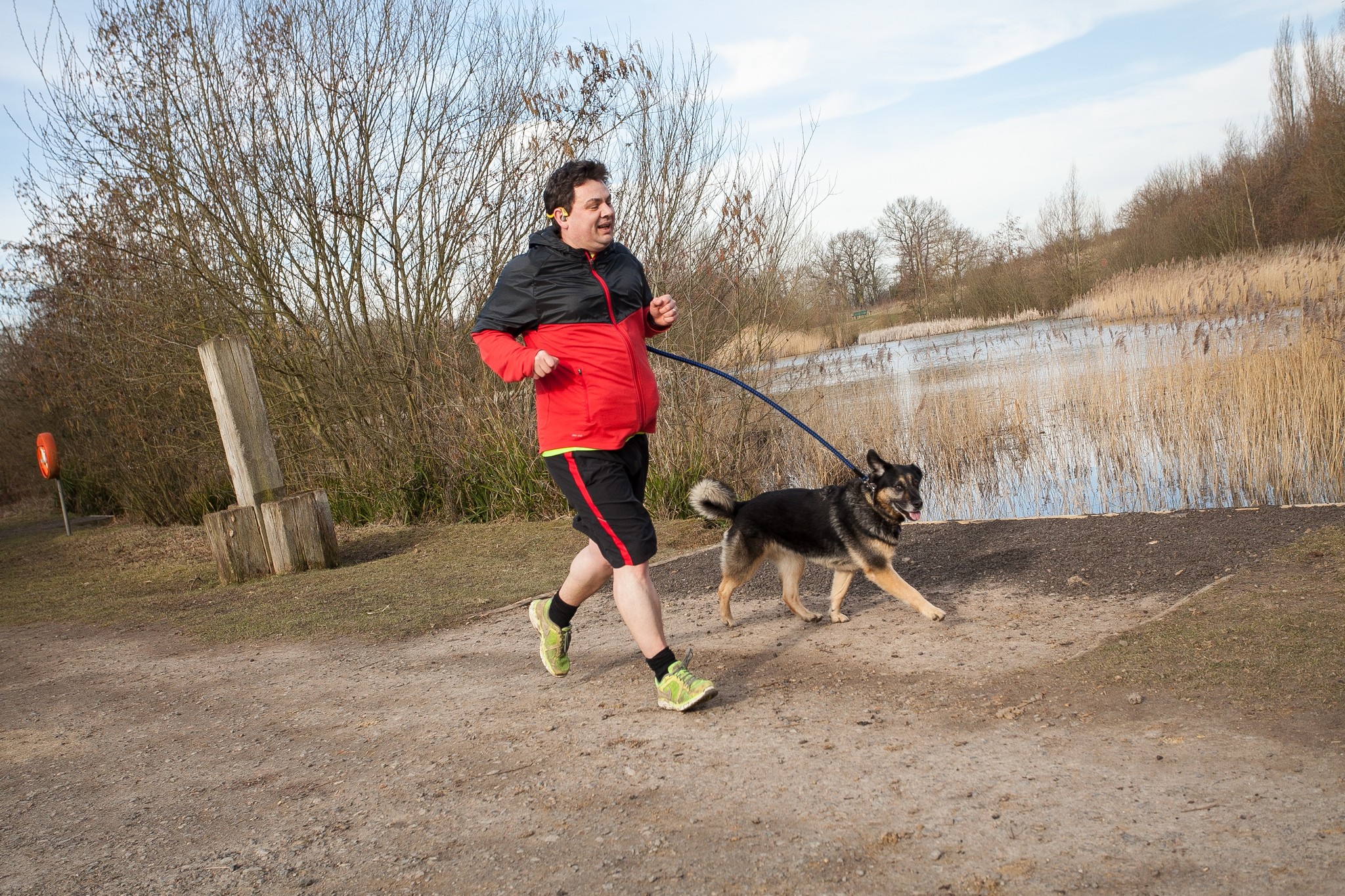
[688,447,946,624]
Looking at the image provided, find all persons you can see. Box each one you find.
[471,160,719,712]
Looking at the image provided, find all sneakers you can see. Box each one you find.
[655,648,717,711]
[528,597,573,677]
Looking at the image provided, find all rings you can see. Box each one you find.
[672,309,676,314]
